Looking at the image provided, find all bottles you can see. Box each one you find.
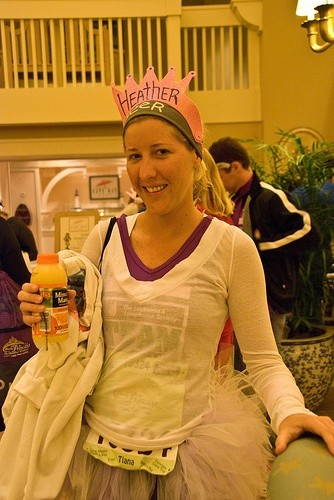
[29,252,69,344]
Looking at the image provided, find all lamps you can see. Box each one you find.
[295,0,334,53]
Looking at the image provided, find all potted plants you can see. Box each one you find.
[248,126,334,413]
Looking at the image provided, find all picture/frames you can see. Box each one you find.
[275,127,327,186]
[89,175,121,200]
[53,210,99,254]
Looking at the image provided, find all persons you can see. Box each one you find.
[208,133,312,418]
[0,198,32,299]
[18,68,334,500]
[7,204,38,261]
[286,165,334,253]
